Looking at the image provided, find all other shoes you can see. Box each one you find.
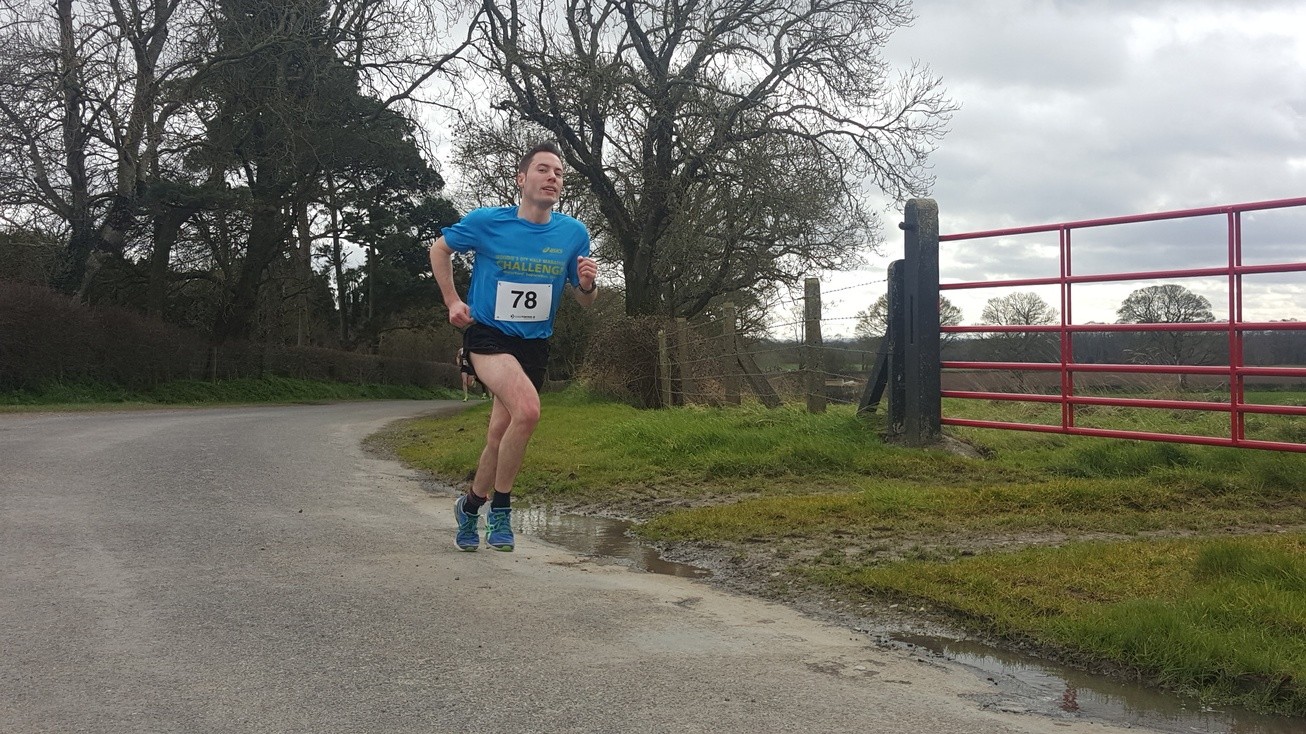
[482,393,486,400]
[473,382,476,388]
[463,397,468,402]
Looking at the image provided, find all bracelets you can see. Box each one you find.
[578,281,596,295]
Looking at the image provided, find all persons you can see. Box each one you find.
[429,143,598,551]
[475,378,488,399]
[456,347,477,402]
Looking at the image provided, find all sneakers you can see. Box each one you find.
[453,494,481,552]
[484,501,514,552]
